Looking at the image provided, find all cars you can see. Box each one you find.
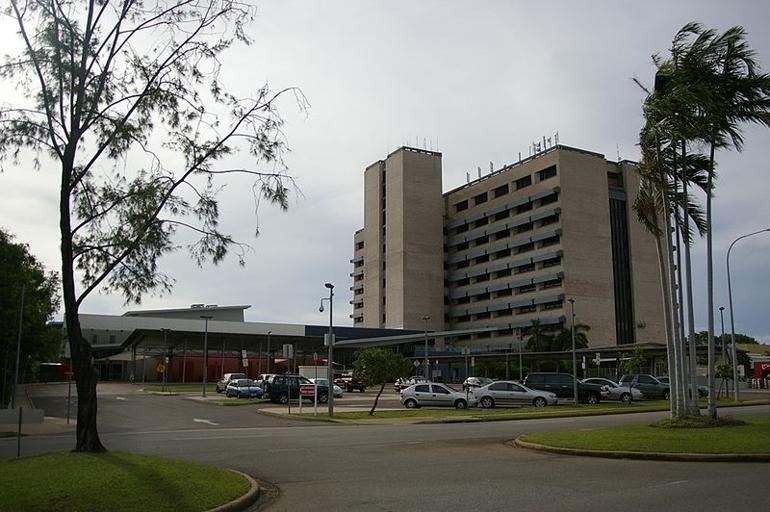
[217,366,366,405]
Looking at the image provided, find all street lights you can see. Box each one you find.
[160,327,171,392]
[725,227,770,401]
[200,314,213,398]
[11,275,40,410]
[266,330,273,371]
[423,313,430,384]
[318,283,334,417]
[567,298,580,406]
[720,305,729,398]
